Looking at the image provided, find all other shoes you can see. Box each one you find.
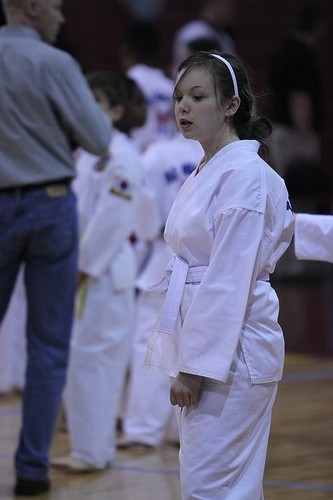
[13,479,51,497]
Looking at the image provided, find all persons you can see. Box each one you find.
[155,49,293,500]
[1,1,332,500]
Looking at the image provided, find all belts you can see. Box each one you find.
[0,179,67,194]
